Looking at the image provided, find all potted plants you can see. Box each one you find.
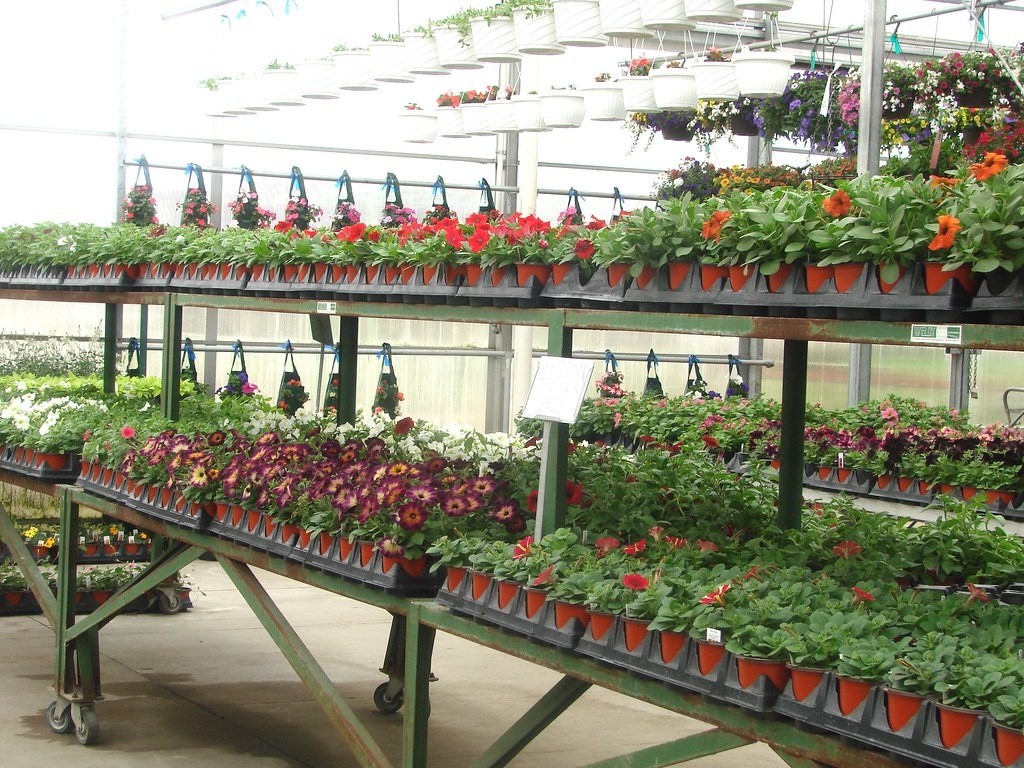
[586,153,1020,316]
[0,555,193,611]
[191,0,796,143]
[768,446,1022,511]
[0,220,575,305]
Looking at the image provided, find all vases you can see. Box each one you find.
[204,490,431,582]
[23,541,152,562]
[77,457,214,528]
[3,442,65,472]
[444,561,1024,768]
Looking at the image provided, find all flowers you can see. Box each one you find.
[0,370,107,431]
[114,154,631,231]
[632,42,1024,196]
[509,516,995,610]
[117,419,534,542]
[11,515,154,547]
[577,371,1023,468]
[124,337,407,420]
